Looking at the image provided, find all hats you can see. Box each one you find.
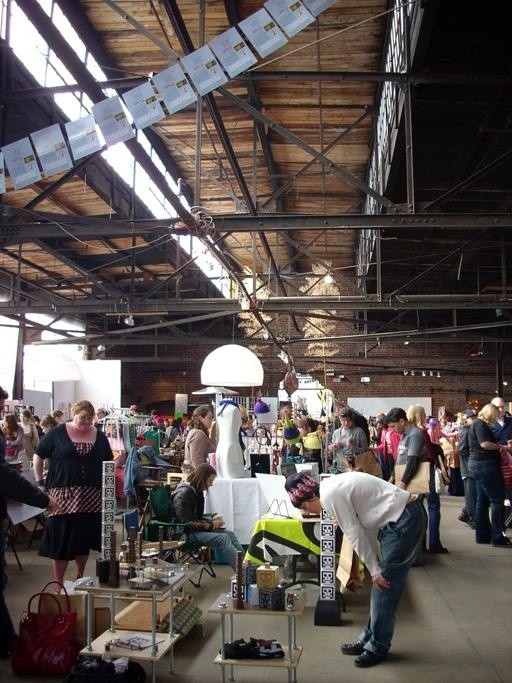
[284,472,319,507]
[338,405,354,421]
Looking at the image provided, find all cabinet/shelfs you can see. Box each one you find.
[74,559,204,682]
[208,590,307,682]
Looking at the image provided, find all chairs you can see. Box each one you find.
[127,444,180,530]
[148,483,219,585]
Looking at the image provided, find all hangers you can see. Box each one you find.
[135,425,155,437]
[98,407,142,424]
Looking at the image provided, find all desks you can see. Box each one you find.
[244,514,366,591]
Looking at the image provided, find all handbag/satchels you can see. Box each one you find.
[10,582,78,676]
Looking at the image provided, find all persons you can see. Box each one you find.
[1,396,115,582]
[130,404,245,570]
[1,384,58,662]
[282,470,428,669]
[297,397,511,567]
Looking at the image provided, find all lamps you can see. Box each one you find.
[402,369,442,377]
[201,280,265,386]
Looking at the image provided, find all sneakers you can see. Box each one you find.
[457,506,511,546]
[341,638,387,667]
